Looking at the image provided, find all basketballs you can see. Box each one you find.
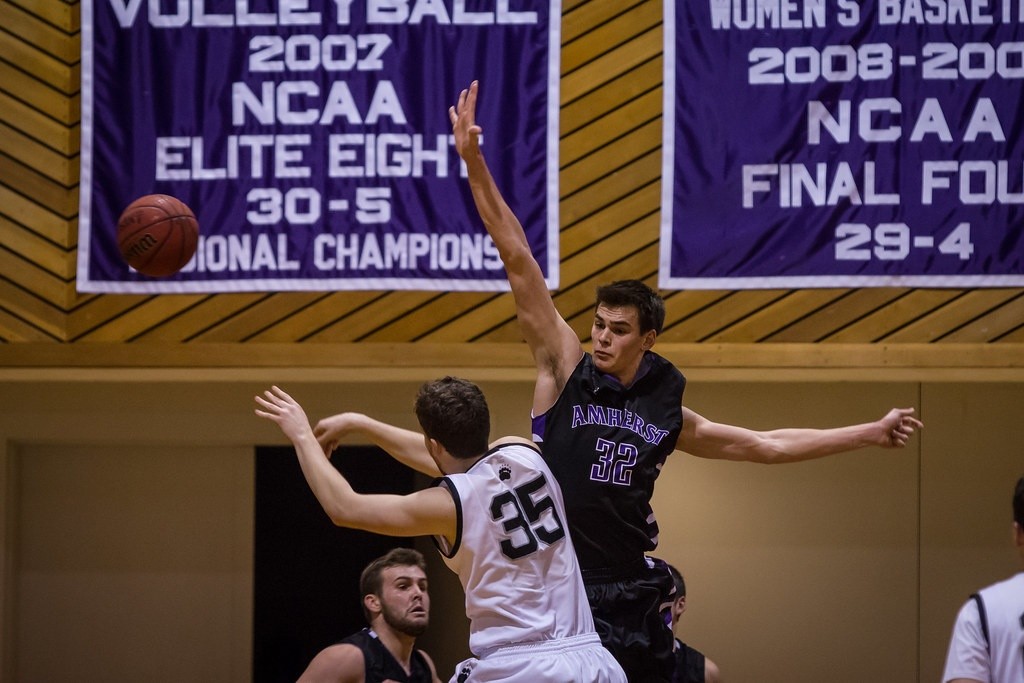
[116,192,201,278]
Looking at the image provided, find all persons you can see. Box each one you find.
[942,477,1024,683]
[295,550,442,683]
[668,564,719,683]
[255,375,629,683]
[450,80,924,683]
